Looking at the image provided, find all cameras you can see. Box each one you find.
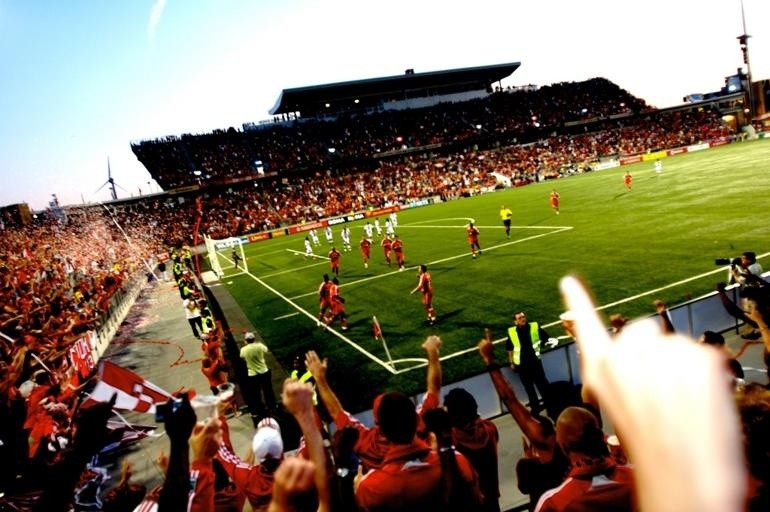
[155,402,181,421]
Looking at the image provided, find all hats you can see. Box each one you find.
[243,331,256,342]
[371,391,418,425]
[251,416,284,463]
